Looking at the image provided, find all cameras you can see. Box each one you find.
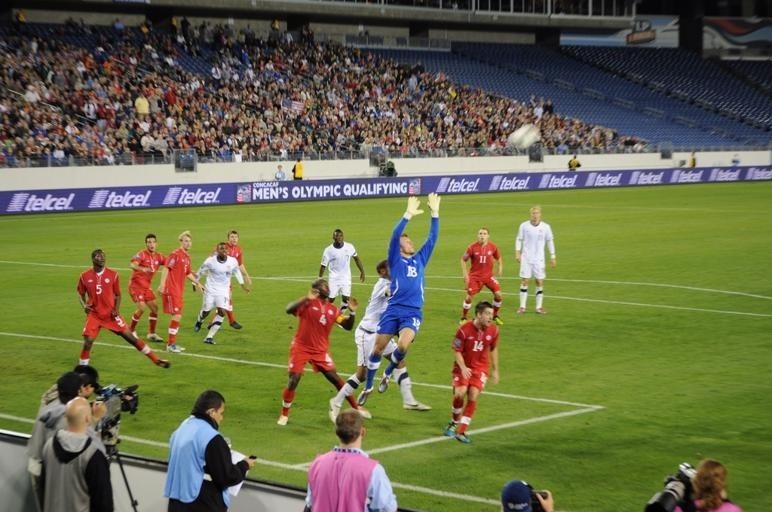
[531,489,548,512]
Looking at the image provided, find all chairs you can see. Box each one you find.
[4,44,772,170]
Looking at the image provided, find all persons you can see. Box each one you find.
[40,373,118,462]
[502,480,554,511]
[379,161,398,177]
[155,231,209,354]
[162,390,256,511]
[75,250,172,369]
[357,193,442,406]
[304,411,398,511]
[444,299,501,442]
[292,158,304,180]
[25,364,102,511]
[512,205,556,314]
[192,243,252,346]
[127,233,174,343]
[213,231,251,329]
[1,13,650,169]
[319,229,365,315]
[275,164,286,182]
[688,150,696,167]
[274,278,371,425]
[460,227,502,325]
[43,397,114,512]
[568,156,581,172]
[671,459,744,511]
[328,259,432,425]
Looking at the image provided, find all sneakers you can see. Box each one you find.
[277,414,289,427]
[459,318,467,325]
[357,388,373,406]
[357,407,371,419]
[157,359,170,368]
[166,343,181,352]
[379,372,391,393]
[444,422,457,436]
[516,307,526,314]
[147,333,163,342]
[404,402,431,410]
[493,316,504,326]
[230,321,242,329]
[203,337,216,344]
[535,308,544,314]
[329,397,341,423]
[455,433,470,443]
[195,321,202,332]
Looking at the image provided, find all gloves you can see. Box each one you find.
[407,196,424,219]
[427,192,441,215]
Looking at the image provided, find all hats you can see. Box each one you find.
[57,372,93,397]
[501,480,532,511]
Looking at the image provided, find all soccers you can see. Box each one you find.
[507,124,540,150]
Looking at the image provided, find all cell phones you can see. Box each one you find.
[248,455,257,460]
